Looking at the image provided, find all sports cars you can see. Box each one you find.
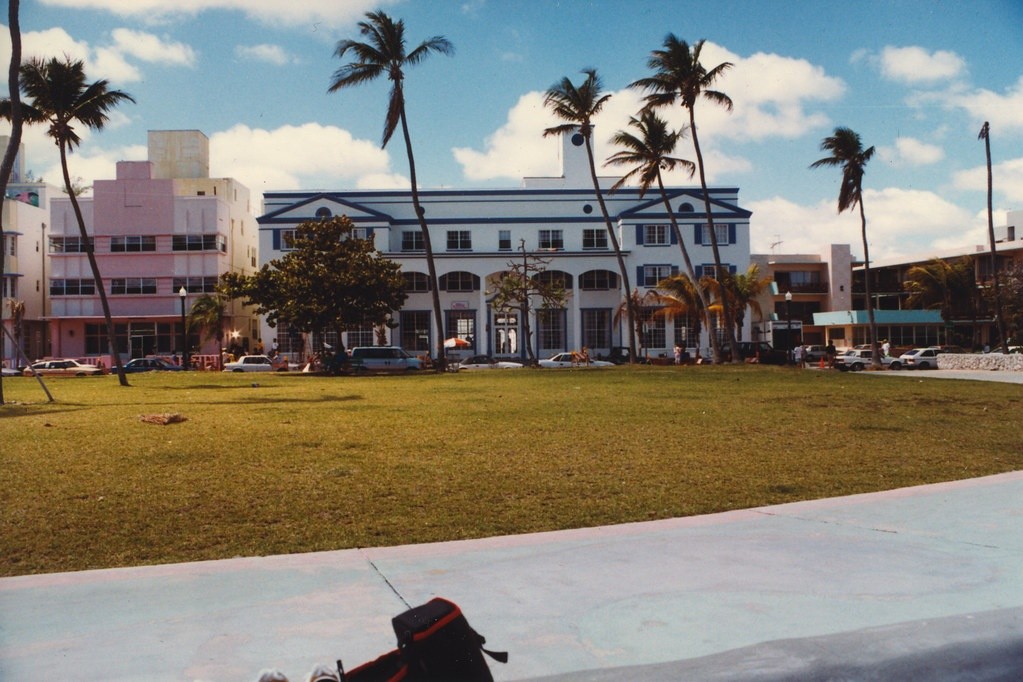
[446,354,525,369]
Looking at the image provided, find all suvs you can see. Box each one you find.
[719,341,787,364]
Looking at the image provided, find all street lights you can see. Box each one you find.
[784,290,793,366]
[179,285,189,370]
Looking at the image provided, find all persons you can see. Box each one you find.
[673,343,682,364]
[824,340,836,368]
[267,338,279,360]
[879,339,891,358]
[254,337,264,354]
[787,343,807,368]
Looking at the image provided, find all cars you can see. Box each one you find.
[989,345,1023,354]
[898,344,971,370]
[110,357,184,374]
[791,345,827,364]
[1,356,96,375]
[832,348,902,371]
[537,352,616,368]
[222,354,300,372]
[854,343,872,349]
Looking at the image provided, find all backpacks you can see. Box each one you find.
[338,596,508,682]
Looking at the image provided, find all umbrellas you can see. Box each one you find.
[443,337,470,352]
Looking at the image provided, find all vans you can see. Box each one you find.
[346,345,427,375]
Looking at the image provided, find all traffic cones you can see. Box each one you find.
[819,355,825,368]
